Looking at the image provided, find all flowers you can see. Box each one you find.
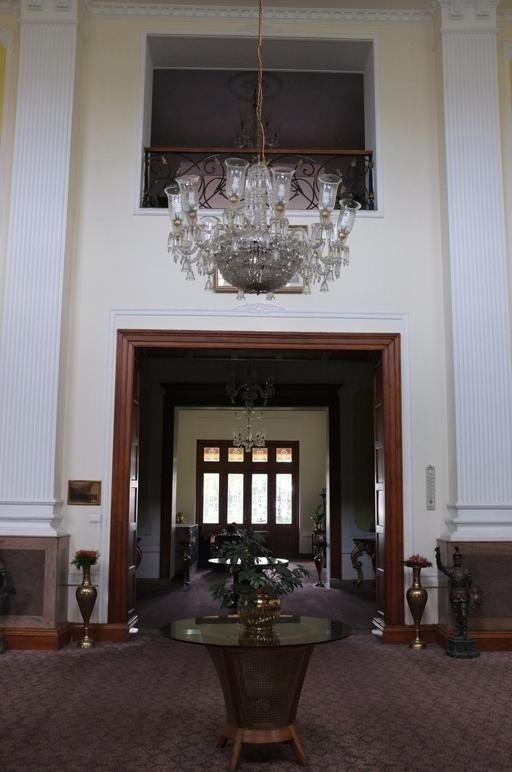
[70,549,103,569]
[211,521,312,609]
[404,554,433,566]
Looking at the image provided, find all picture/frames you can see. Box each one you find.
[68,480,101,505]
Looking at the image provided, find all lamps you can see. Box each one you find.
[226,376,275,453]
[164,1,362,302]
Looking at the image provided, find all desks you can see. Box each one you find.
[210,557,290,608]
[162,614,354,772]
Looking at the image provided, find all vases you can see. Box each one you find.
[406,566,428,649]
[238,594,282,645]
[76,566,97,649]
